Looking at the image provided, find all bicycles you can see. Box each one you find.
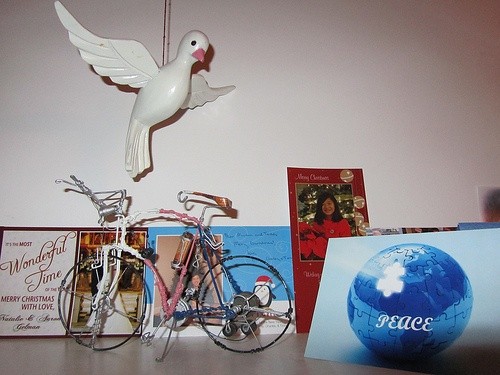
[55,174,293,362]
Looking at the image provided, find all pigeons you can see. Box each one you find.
[53,0,236,180]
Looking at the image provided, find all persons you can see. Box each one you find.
[314,191,353,238]
